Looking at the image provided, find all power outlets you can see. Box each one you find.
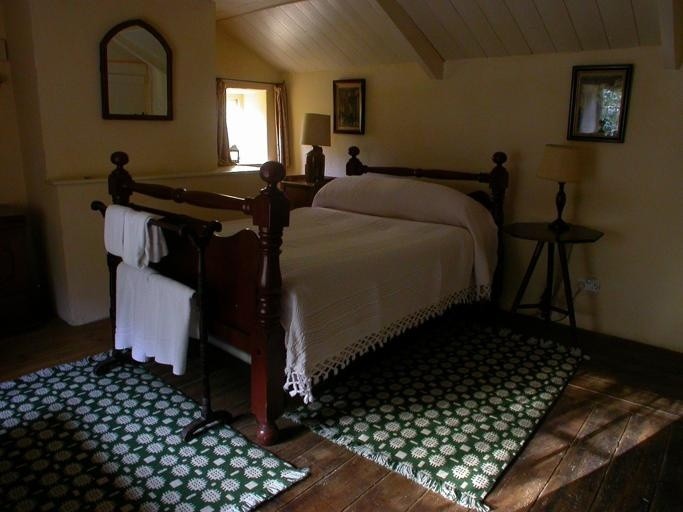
[574,278,600,292]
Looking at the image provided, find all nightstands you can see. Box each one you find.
[275,174,335,211]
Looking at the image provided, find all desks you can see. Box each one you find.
[500,219,607,342]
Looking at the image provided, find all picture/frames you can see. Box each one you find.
[331,77,366,135]
[565,63,634,143]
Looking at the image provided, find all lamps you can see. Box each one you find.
[298,112,332,187]
[533,142,586,232]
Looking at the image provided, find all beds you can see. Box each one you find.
[104,145,516,445]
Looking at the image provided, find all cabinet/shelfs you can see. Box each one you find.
[0,214,30,336]
[89,201,236,447]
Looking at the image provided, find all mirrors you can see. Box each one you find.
[97,16,175,122]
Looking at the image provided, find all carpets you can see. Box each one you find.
[0,340,313,511]
[278,312,587,511]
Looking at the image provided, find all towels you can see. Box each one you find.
[102,202,204,377]
